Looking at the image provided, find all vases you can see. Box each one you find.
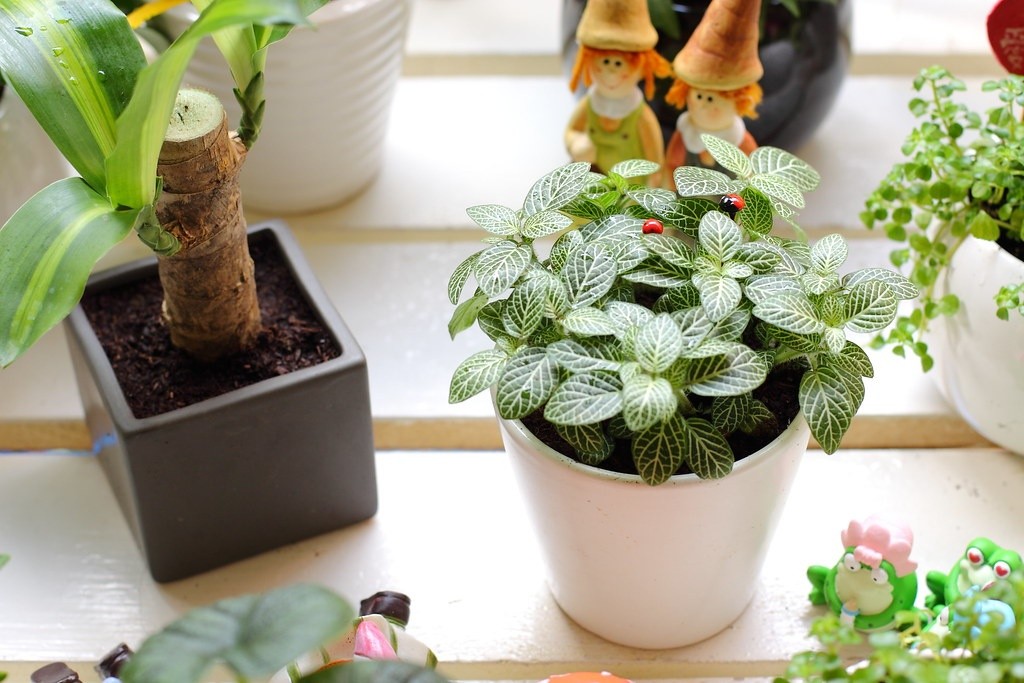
[145,0,412,218]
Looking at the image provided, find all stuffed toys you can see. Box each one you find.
[664,0,764,191]
[563,0,675,192]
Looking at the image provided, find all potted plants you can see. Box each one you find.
[858,64,1024,454]
[448,137,919,650]
[558,0,854,179]
[0,0,378,587]
